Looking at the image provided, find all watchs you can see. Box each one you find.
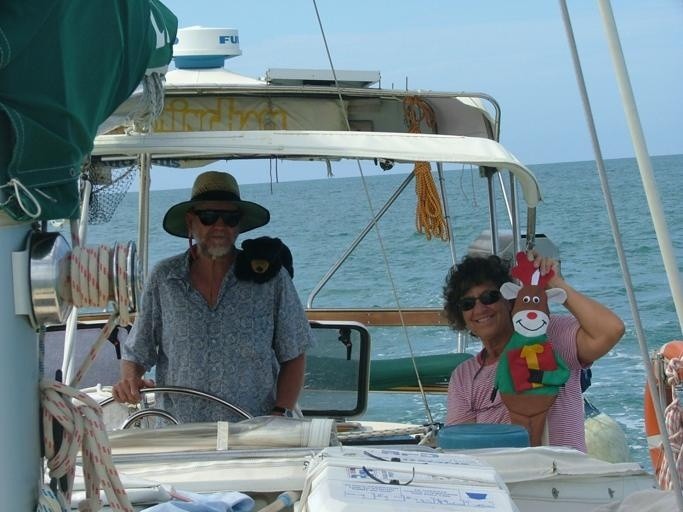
[272,406,293,417]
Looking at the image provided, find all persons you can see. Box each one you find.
[439,250,626,454]
[109,171,316,430]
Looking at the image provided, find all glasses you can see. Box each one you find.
[456,289,501,312]
[191,207,243,228]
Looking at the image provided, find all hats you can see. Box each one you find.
[162,170,271,239]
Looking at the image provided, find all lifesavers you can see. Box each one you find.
[645,341,683,491]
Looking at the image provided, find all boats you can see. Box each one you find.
[0,3,683,510]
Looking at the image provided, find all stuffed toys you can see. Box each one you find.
[233,234,295,284]
[489,252,570,447]
